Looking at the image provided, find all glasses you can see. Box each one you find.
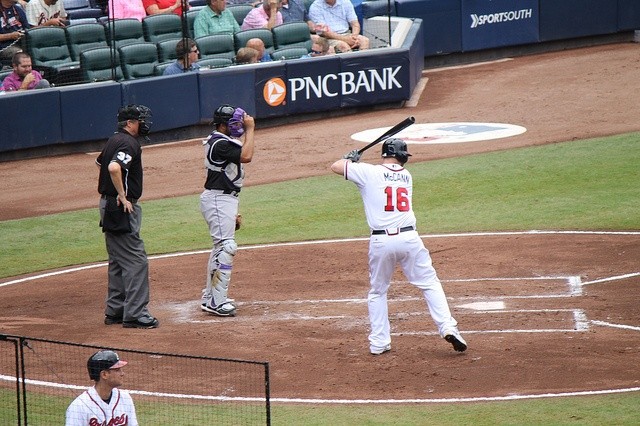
[192,49,199,53]
[311,48,322,54]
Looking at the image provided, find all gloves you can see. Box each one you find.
[344,149,363,162]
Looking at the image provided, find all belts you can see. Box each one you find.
[372,226,414,234]
[205,188,239,196]
[101,192,136,206]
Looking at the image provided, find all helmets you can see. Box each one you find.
[382,138,413,163]
[210,104,247,138]
[87,350,129,380]
[117,104,152,142]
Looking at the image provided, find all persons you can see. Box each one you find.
[2,53,52,92]
[330,137,468,355]
[142,0,192,16]
[280,0,337,54]
[250,0,289,10]
[246,37,272,63]
[162,38,203,75]
[193,0,242,39]
[198,104,256,317]
[94,102,161,330]
[308,38,330,57]
[309,0,370,54]
[232,48,258,65]
[240,0,283,30]
[107,0,147,23]
[66,349,140,425]
[0,0,29,66]
[25,0,68,28]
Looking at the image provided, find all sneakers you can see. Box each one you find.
[201,298,236,311]
[371,344,393,354]
[123,314,159,330]
[207,302,237,317]
[104,313,123,325]
[443,330,468,352]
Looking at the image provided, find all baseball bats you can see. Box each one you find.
[357,116,416,154]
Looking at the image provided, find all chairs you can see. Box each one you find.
[155,62,173,75]
[304,0,315,9]
[118,42,159,79]
[105,18,146,49]
[66,24,108,64]
[0,69,14,81]
[185,10,202,39]
[25,27,80,74]
[144,13,183,43]
[270,46,309,61]
[271,21,313,49]
[234,28,276,55]
[227,4,253,25]
[60,0,108,20]
[157,39,183,62]
[195,58,233,68]
[195,31,236,61]
[79,46,125,81]
